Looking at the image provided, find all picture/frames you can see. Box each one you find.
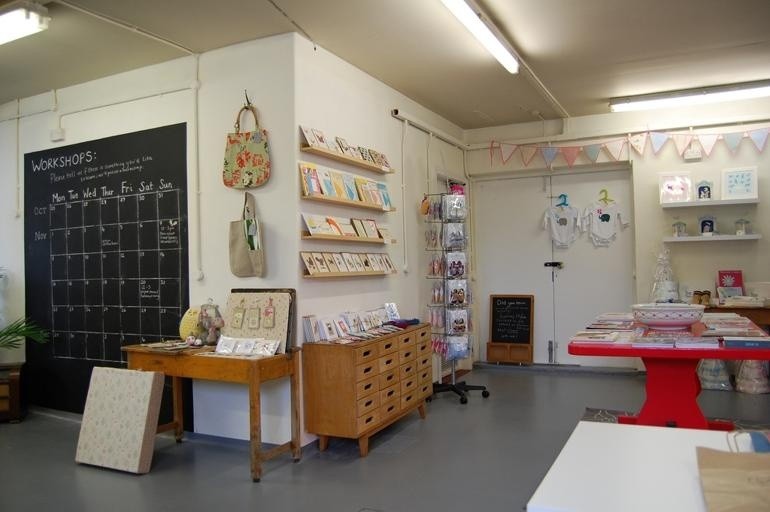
[660,171,692,203]
[721,166,758,200]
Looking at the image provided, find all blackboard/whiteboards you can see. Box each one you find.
[24,122,194,434]
[489,294,533,343]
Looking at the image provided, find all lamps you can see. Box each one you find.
[0,1,52,44]
[608,76,769,113]
[435,0,520,75]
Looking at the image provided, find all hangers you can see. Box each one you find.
[555,190,613,208]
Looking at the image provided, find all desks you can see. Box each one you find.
[121,341,301,482]
[525,420,770,512]
[567,312,769,433]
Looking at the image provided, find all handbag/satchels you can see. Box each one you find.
[222,129,271,189]
[228,219,266,278]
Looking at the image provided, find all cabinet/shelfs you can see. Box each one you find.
[300,143,396,278]
[662,198,760,242]
[302,323,432,458]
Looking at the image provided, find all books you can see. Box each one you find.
[297,124,408,346]
[572,310,770,350]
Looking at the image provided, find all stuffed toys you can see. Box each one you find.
[185,304,225,346]
